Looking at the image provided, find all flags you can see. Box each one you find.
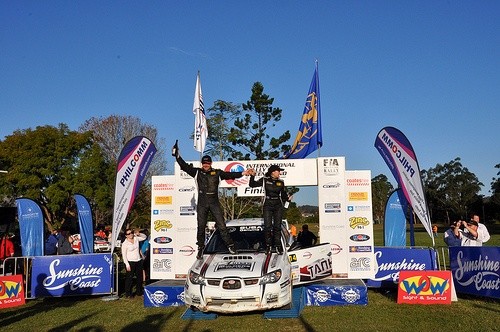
[279,66,323,159]
[192,74,208,153]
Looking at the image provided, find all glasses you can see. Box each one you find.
[126,232,134,235]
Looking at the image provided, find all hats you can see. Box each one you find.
[202,155,212,162]
[265,165,285,177]
[467,220,479,227]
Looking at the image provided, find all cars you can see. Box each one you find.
[68,233,110,253]
[184,217,332,314]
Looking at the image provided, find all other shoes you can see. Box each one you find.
[227,243,239,255]
[265,245,272,254]
[196,247,204,260]
[276,246,283,255]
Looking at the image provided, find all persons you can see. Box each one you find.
[121,229,151,298]
[175,154,250,259]
[445,214,491,247]
[296,224,317,248]
[0,228,113,264]
[249,164,291,256]
